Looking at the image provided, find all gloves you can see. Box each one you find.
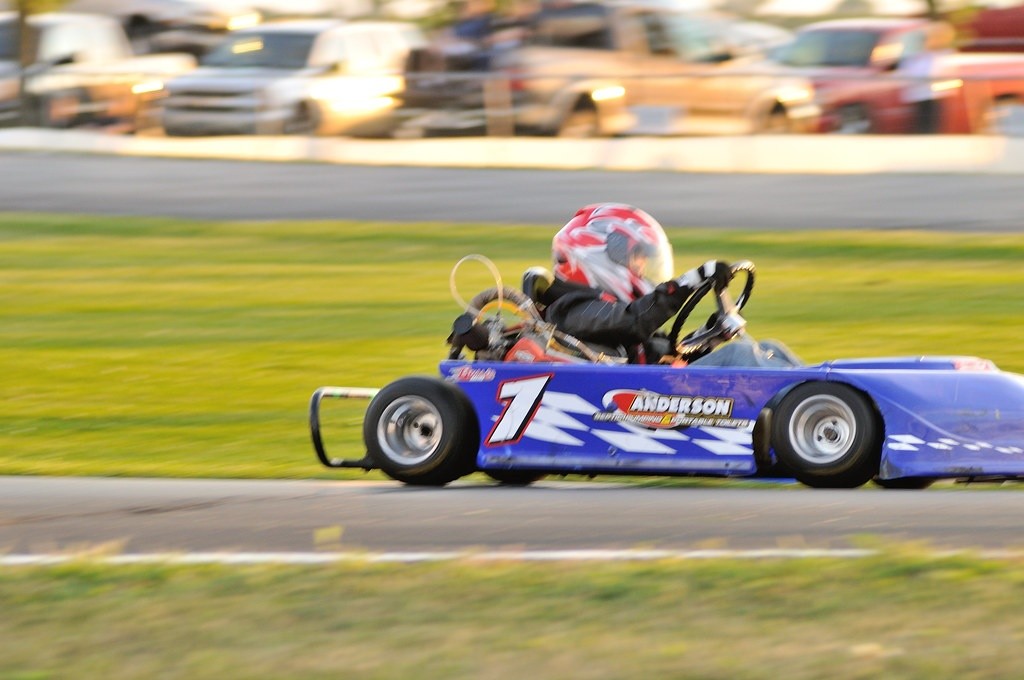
[697,314,722,354]
[679,258,736,297]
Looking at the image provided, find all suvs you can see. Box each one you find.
[164,16,427,142]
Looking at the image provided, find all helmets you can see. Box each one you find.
[549,203,674,309]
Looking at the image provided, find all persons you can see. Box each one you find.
[544,198,726,362]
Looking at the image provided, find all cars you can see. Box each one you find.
[788,14,961,138]
[1,10,130,132]
[411,6,791,133]
